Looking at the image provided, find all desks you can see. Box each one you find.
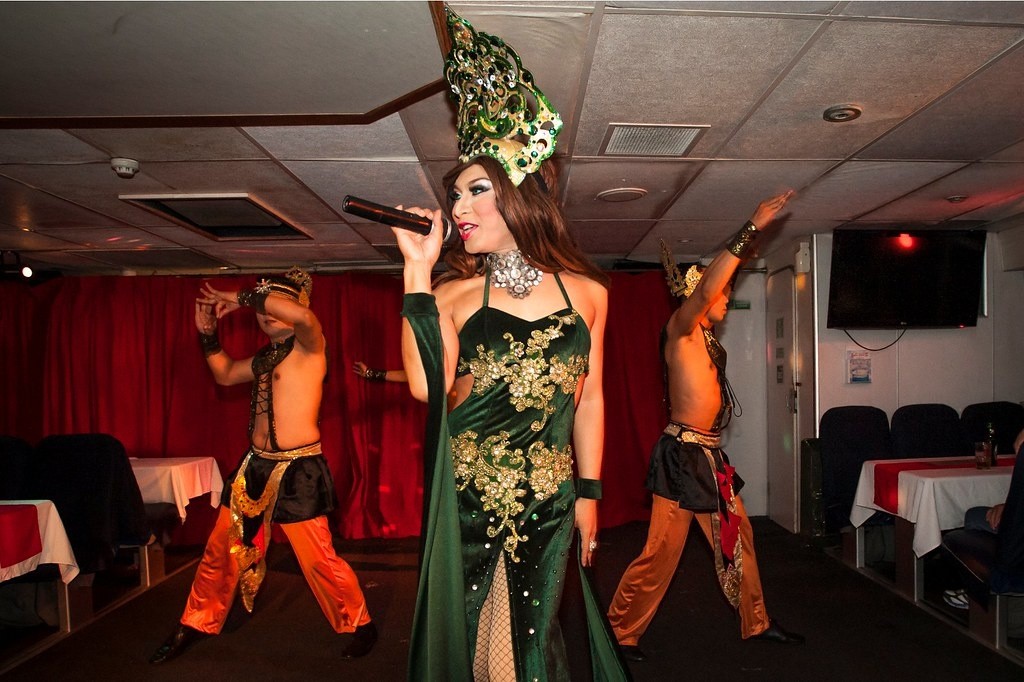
[848,455,1019,599]
[120,456,224,587]
[0,498,79,632]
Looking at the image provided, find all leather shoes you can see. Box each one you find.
[149,622,212,664]
[339,622,379,660]
[748,618,805,647]
[620,644,647,662]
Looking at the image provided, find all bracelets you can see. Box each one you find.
[575,477,602,499]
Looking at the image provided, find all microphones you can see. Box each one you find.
[342,196,459,245]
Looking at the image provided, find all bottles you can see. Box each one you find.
[985,429,997,466]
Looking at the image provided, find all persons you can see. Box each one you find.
[150,260,380,658]
[353,156,630,682]
[607,188,804,661]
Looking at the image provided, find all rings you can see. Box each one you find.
[590,540,598,551]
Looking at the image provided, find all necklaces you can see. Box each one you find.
[484,248,543,299]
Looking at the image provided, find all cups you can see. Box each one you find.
[976,442,992,470]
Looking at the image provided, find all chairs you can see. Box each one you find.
[891,405,963,461]
[819,405,891,567]
[939,447,1024,650]
[962,402,1023,455]
[0,436,180,589]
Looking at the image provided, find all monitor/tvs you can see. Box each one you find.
[826,228,986,330]
